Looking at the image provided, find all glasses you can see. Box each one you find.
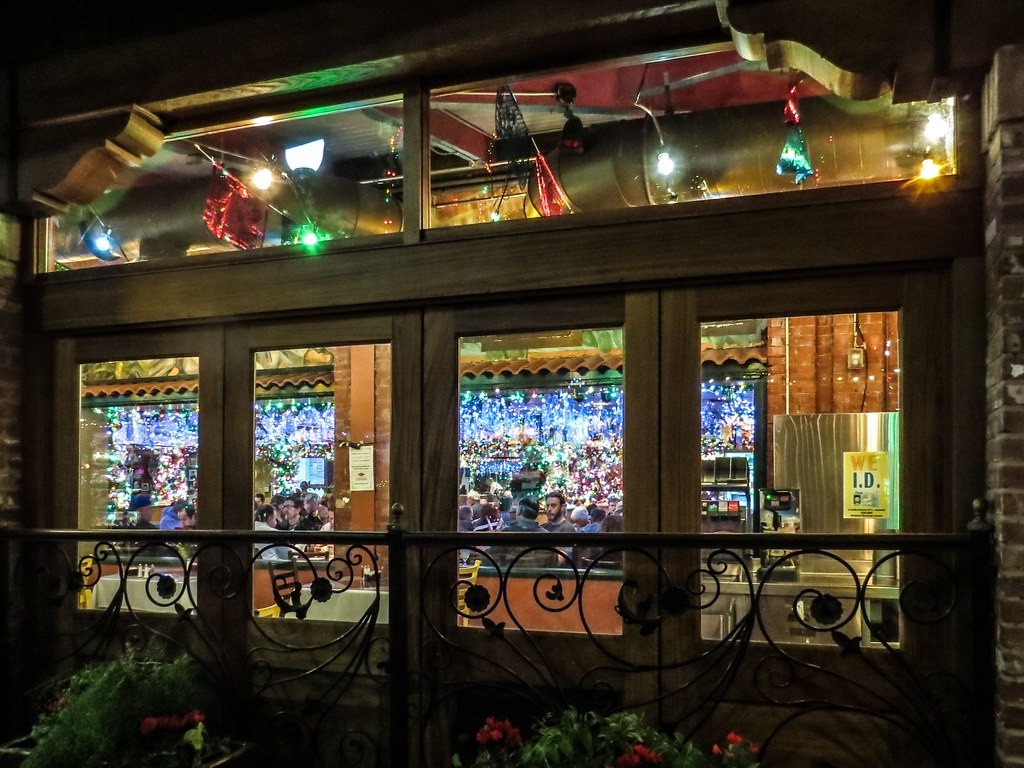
[281,504,295,509]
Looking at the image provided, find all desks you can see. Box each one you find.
[284,576,389,623]
[93,570,197,614]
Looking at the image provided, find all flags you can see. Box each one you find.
[536,152,564,217]
[784,83,803,124]
[775,125,814,184]
[202,161,270,250]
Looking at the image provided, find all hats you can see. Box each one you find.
[128,496,150,510]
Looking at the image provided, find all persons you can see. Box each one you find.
[111,496,199,547]
[253,481,334,560]
[457,492,622,568]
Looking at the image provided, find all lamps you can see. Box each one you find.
[285,138,324,179]
[95,226,114,250]
[559,106,586,153]
[633,62,674,176]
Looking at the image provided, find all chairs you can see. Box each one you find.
[78,588,92,609]
[458,559,481,626]
[78,553,93,590]
[254,599,288,618]
